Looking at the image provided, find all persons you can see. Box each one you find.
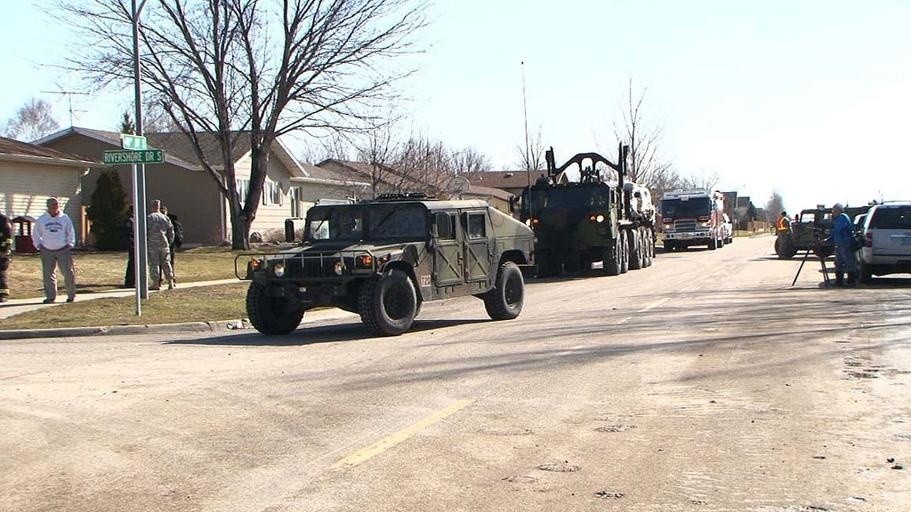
[158,204,183,288]
[119,203,137,286]
[773,209,790,235]
[29,197,77,304]
[0,211,15,305]
[145,198,175,291]
[816,202,860,289]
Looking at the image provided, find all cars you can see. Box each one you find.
[854,204,911,284]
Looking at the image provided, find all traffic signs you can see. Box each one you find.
[102,149,164,165]
[120,134,147,151]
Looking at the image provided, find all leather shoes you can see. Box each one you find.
[0,297,8,302]
[43,296,76,303]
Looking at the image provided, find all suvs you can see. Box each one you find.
[774,205,872,259]
[233,192,535,337]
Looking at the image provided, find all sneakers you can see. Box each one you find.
[148,283,177,290]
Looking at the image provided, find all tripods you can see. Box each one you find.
[791,231,831,288]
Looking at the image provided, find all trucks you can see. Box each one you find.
[658,189,732,251]
[509,140,657,278]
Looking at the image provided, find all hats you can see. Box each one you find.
[47,197,58,207]
[150,200,160,205]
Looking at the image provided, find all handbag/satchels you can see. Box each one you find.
[848,235,864,250]
